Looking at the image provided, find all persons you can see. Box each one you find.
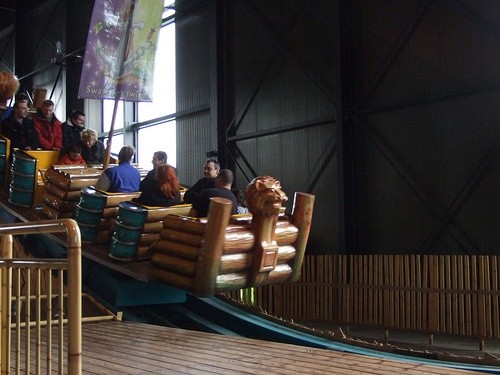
[0,94,180,207]
[183,159,221,204]
[188,169,239,217]
